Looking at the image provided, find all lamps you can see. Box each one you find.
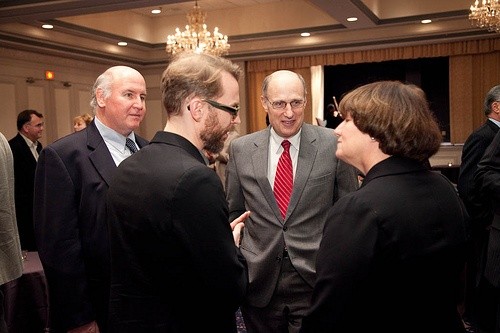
[165,1,231,56]
[469,0,500,32]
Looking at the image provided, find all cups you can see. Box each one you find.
[21,250,28,262]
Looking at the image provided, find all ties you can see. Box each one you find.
[125,138,139,155]
[274,140,293,220]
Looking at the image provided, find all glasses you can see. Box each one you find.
[187,97,240,121]
[264,96,306,110]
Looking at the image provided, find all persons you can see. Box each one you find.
[458,84,500,333]
[73,113,93,132]
[226,70,359,333]
[1,110,44,333]
[86,50,251,333]
[300,80,476,333]
[33,66,151,333]
